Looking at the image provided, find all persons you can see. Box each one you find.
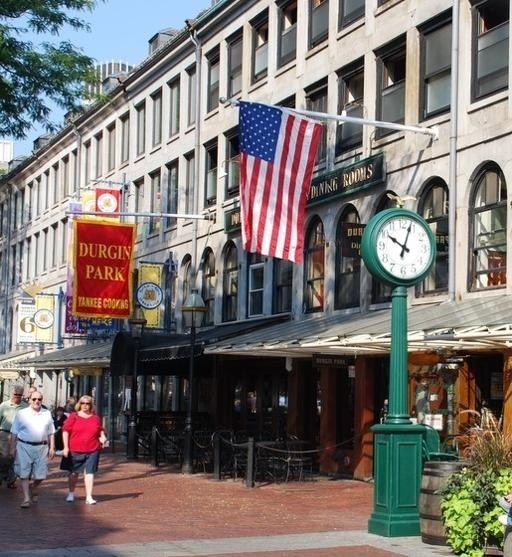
[0,383,111,507]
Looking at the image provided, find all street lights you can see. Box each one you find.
[181,289,207,474]
[127,302,147,462]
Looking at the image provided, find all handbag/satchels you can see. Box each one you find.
[60,454,74,472]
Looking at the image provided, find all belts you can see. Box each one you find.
[18,438,47,445]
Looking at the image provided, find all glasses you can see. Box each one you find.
[33,398,42,401]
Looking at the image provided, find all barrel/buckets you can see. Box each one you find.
[419,461,476,546]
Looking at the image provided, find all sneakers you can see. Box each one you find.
[66,495,74,502]
[85,498,96,505]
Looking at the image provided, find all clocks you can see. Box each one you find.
[363,205,440,539]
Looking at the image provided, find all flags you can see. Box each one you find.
[71,217,138,319]
[236,100,325,267]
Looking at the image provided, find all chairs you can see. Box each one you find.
[133,417,317,487]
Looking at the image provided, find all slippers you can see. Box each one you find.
[20,501,31,508]
